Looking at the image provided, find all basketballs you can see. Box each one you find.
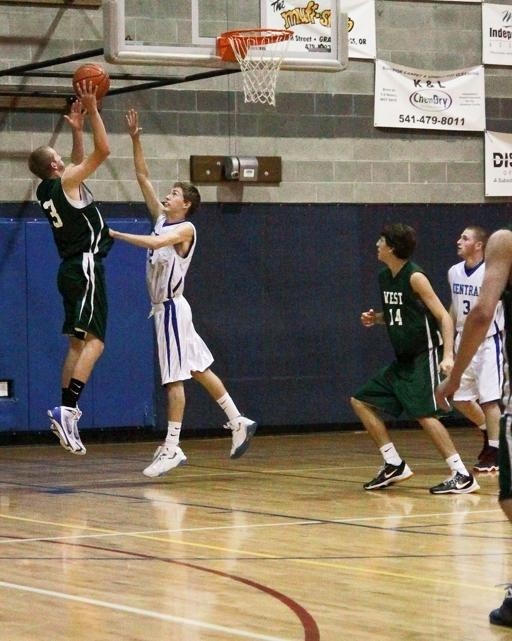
[73,64,110,100]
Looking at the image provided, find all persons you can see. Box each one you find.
[448,224,505,472]
[434,223,512,627]
[350,224,481,494]
[29,79,114,456]
[108,109,257,478]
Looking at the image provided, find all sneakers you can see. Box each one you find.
[223,415,258,458]
[473,446,501,471]
[430,470,481,493]
[490,582,512,627]
[363,459,414,490]
[142,445,187,477]
[479,428,488,459]
[46,405,77,453]
[48,411,86,456]
